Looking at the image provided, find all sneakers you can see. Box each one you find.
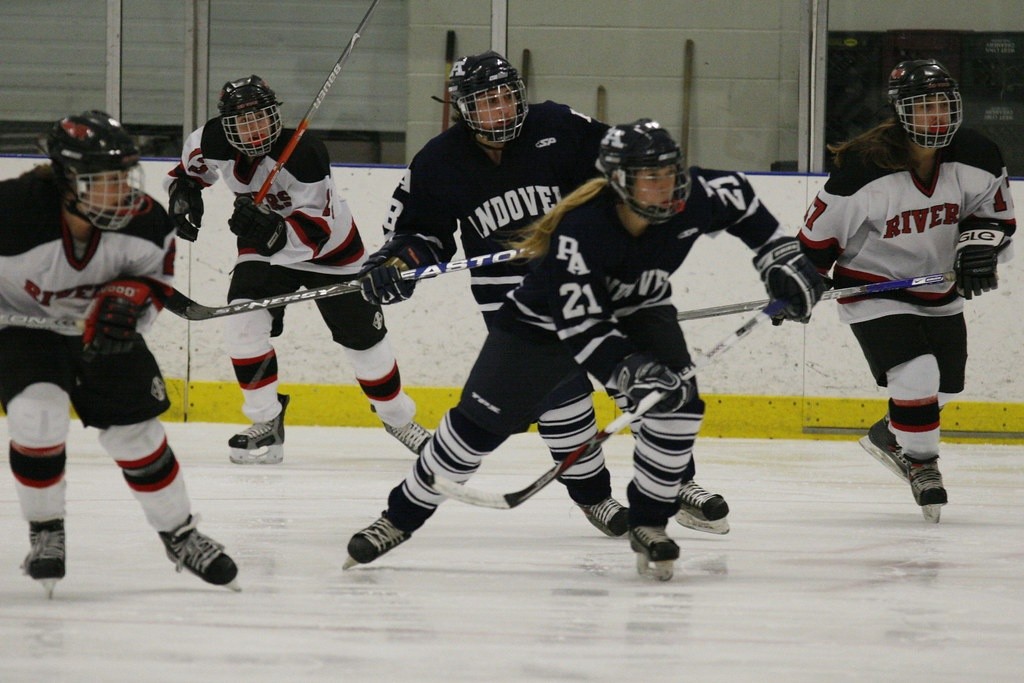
[629,524,681,582]
[24,512,66,601]
[228,393,290,466]
[341,509,412,570]
[858,414,911,486]
[577,495,630,539]
[383,421,434,455]
[157,514,240,592]
[903,451,948,524]
[675,479,730,535]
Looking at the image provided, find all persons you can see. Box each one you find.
[342,115,823,579]
[166,75,432,466]
[0,109,244,598]
[796,56,1017,522]
[348,51,733,539]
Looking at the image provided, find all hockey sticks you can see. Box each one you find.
[429,301,780,511]
[0,303,87,330]
[251,0,380,206]
[679,264,954,323]
[152,246,531,321]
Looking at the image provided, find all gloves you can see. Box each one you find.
[752,237,822,321]
[79,279,150,368]
[604,351,695,415]
[168,177,204,243]
[355,235,431,306]
[952,220,1005,301]
[772,271,834,327]
[227,196,287,257]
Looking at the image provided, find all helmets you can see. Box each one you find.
[448,51,529,143]
[599,116,691,224]
[888,59,963,149]
[217,75,283,158]
[46,108,144,230]
